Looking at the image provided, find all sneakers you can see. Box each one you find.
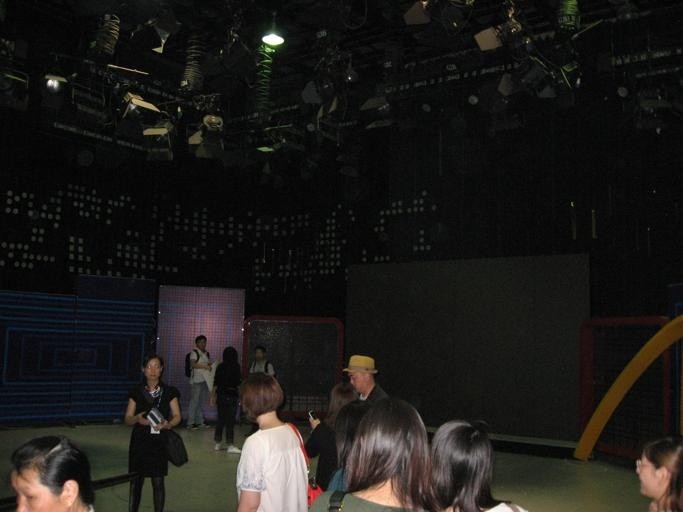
[214,441,242,455]
[186,422,212,430]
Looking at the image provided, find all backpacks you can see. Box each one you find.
[184,348,200,377]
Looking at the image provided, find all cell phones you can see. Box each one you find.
[308,408,318,421]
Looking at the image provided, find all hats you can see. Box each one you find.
[342,355,379,374]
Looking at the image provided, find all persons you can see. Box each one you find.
[121,354,189,512]
[209,347,241,454]
[632,432,682,512]
[231,373,312,512]
[243,344,277,437]
[185,334,210,430]
[304,353,528,512]
[6,434,93,511]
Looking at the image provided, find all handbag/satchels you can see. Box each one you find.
[161,428,189,467]
[306,481,325,508]
